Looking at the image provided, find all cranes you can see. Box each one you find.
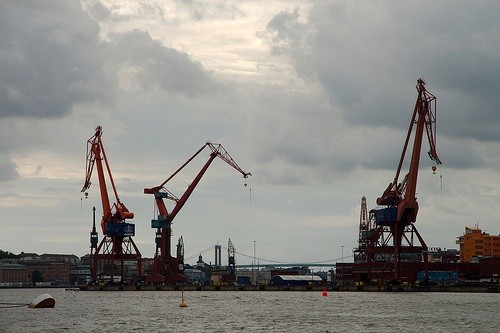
[143,142,252,289]
[352,77,443,292]
[80,125,143,287]
